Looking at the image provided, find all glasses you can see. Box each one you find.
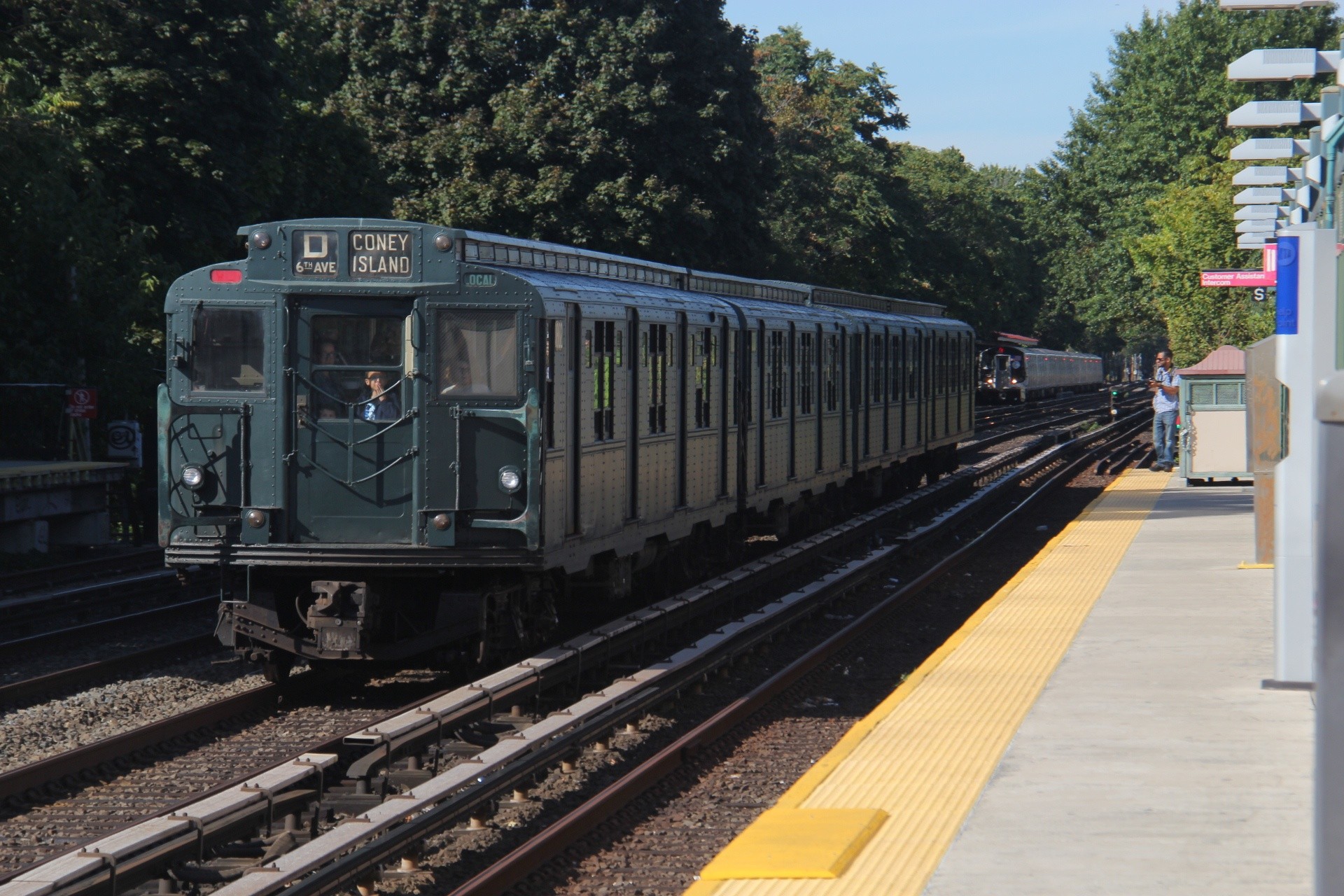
[370,375,386,380]
[319,351,335,358]
[1155,358,1164,361]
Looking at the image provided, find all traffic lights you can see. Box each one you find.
[1110,387,1120,415]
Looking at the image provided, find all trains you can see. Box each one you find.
[154,217,975,686]
[975,330,1103,403]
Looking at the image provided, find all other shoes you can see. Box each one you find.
[1164,463,1171,472]
[1150,464,1165,471]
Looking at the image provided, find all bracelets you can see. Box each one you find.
[1157,383,1165,389]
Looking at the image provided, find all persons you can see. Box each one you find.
[305,339,356,421]
[438,354,492,396]
[354,369,404,422]
[313,403,337,421]
[1147,347,1181,473]
